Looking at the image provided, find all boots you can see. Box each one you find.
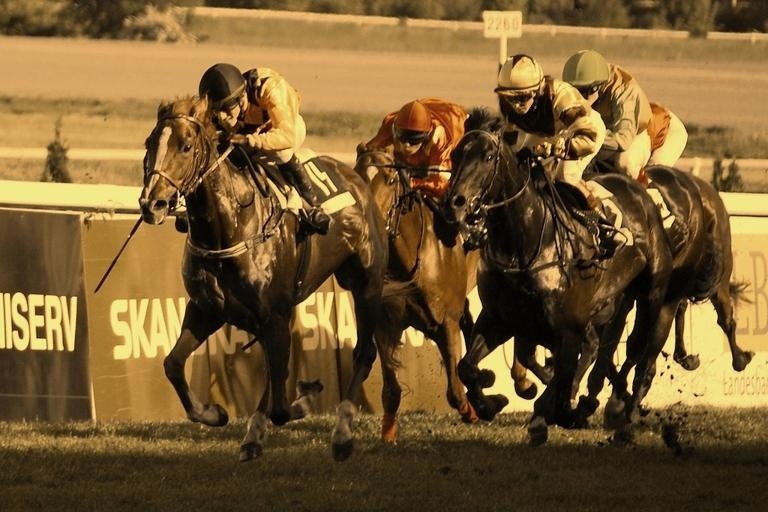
[583,196,626,263]
[276,154,331,227]
[646,182,688,249]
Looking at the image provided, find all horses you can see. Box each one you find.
[139,93,389,463]
[350,106,755,447]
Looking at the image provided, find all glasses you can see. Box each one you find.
[577,82,602,96]
[503,94,533,104]
[396,129,426,144]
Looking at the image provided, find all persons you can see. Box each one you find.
[174,62,330,235]
[493,54,617,243]
[366,99,470,195]
[563,51,687,229]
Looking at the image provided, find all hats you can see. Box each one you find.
[562,50,609,85]
[199,63,249,108]
[395,100,432,132]
[494,54,546,96]
